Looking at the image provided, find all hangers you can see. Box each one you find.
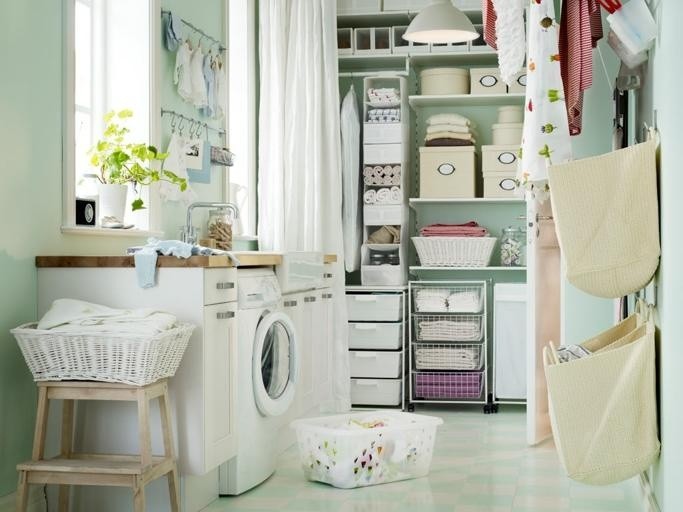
[182,24,216,55]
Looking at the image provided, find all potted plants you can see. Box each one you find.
[89,107,188,231]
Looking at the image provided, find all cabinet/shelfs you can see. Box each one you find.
[36,266,238,475]
[14,377,180,512]
[336,0,530,413]
[276,262,353,452]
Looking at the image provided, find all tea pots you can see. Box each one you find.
[230,183,249,235]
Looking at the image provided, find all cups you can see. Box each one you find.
[605,1,657,70]
[247,239,258,251]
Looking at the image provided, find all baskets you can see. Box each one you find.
[409,234,498,269]
[8,319,198,389]
[287,409,445,489]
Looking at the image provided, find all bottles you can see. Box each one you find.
[206,207,231,250]
[497,227,527,267]
[207,234,216,250]
[371,254,397,266]
[80,173,100,226]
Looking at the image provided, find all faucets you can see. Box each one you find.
[178,201,239,246]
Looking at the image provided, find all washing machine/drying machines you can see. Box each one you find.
[219,266,299,496]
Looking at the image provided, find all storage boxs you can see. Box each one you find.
[482,172,523,199]
[481,143,520,171]
[491,123,522,145]
[508,66,526,94]
[469,67,508,94]
[418,145,476,200]
[420,68,468,95]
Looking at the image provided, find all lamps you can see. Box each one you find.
[401,0,481,44]
